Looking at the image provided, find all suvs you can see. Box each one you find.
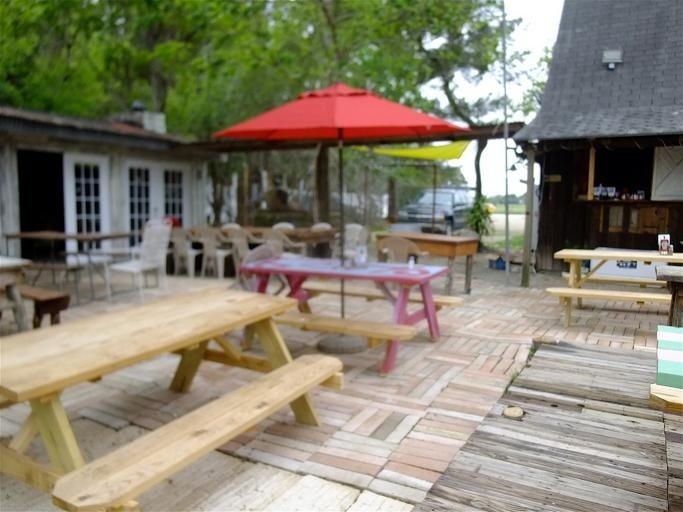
[393,182,493,235]
